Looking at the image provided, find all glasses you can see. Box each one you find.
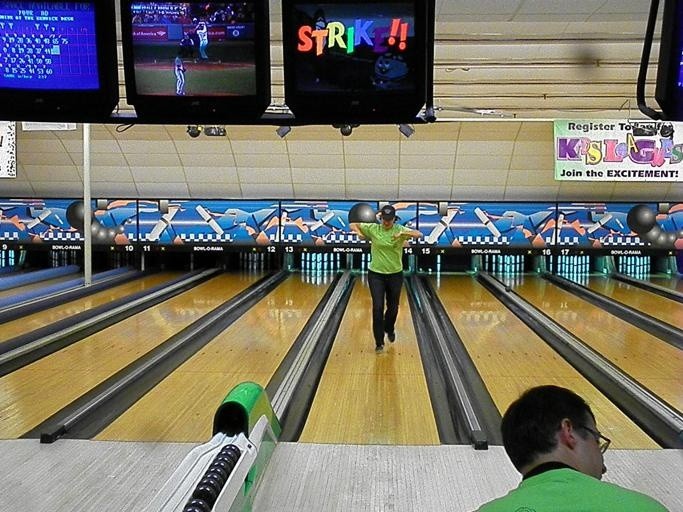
[578,421,611,455]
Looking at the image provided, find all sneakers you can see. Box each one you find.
[387,330,396,343]
[375,342,385,352]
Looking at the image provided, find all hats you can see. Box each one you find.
[380,204,395,221]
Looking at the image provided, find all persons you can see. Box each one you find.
[174,51,187,96]
[349,205,424,357]
[132,2,255,65]
[473,384,670,512]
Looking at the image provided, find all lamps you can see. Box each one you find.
[396,123,414,141]
[185,124,197,138]
[200,125,231,138]
[274,126,295,137]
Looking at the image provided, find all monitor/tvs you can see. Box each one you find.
[655,0,683,120]
[120,0,271,119]
[281,0,436,125]
[0,0,119,121]
